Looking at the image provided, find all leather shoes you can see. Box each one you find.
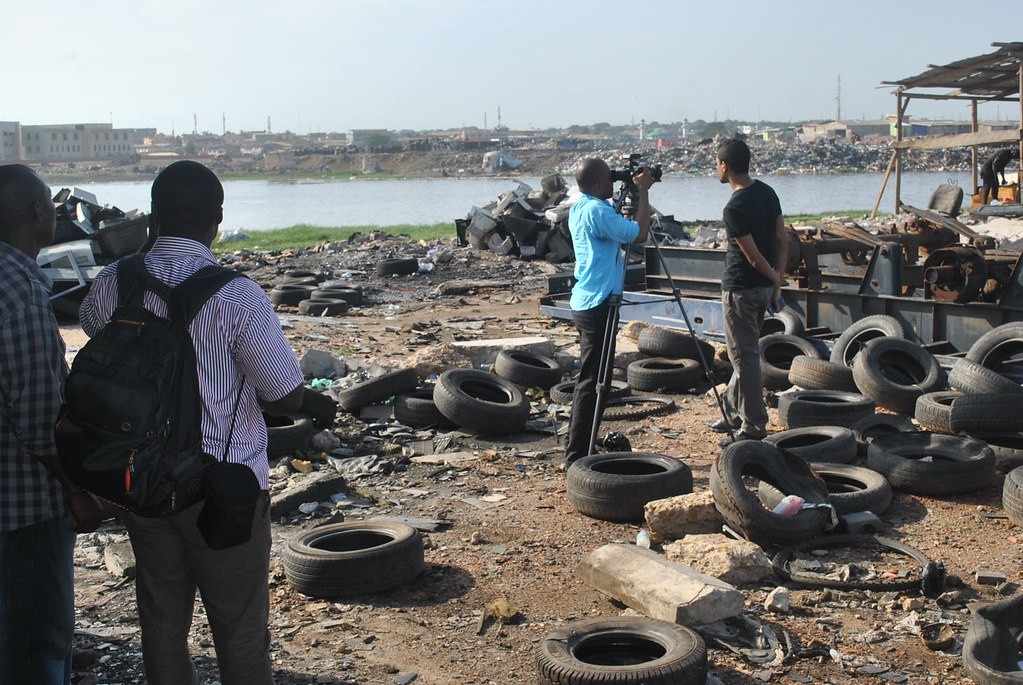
[716,428,754,448]
[707,414,743,432]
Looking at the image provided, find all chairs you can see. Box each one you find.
[908,184,964,217]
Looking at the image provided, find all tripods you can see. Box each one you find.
[588,205,737,457]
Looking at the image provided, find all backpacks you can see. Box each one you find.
[52,252,251,519]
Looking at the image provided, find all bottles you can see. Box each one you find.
[637,530,650,548]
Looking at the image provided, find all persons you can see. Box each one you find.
[981,149,1019,205]
[0,163,104,685]
[80,159,304,685]
[707,138,787,447]
[565,158,651,463]
